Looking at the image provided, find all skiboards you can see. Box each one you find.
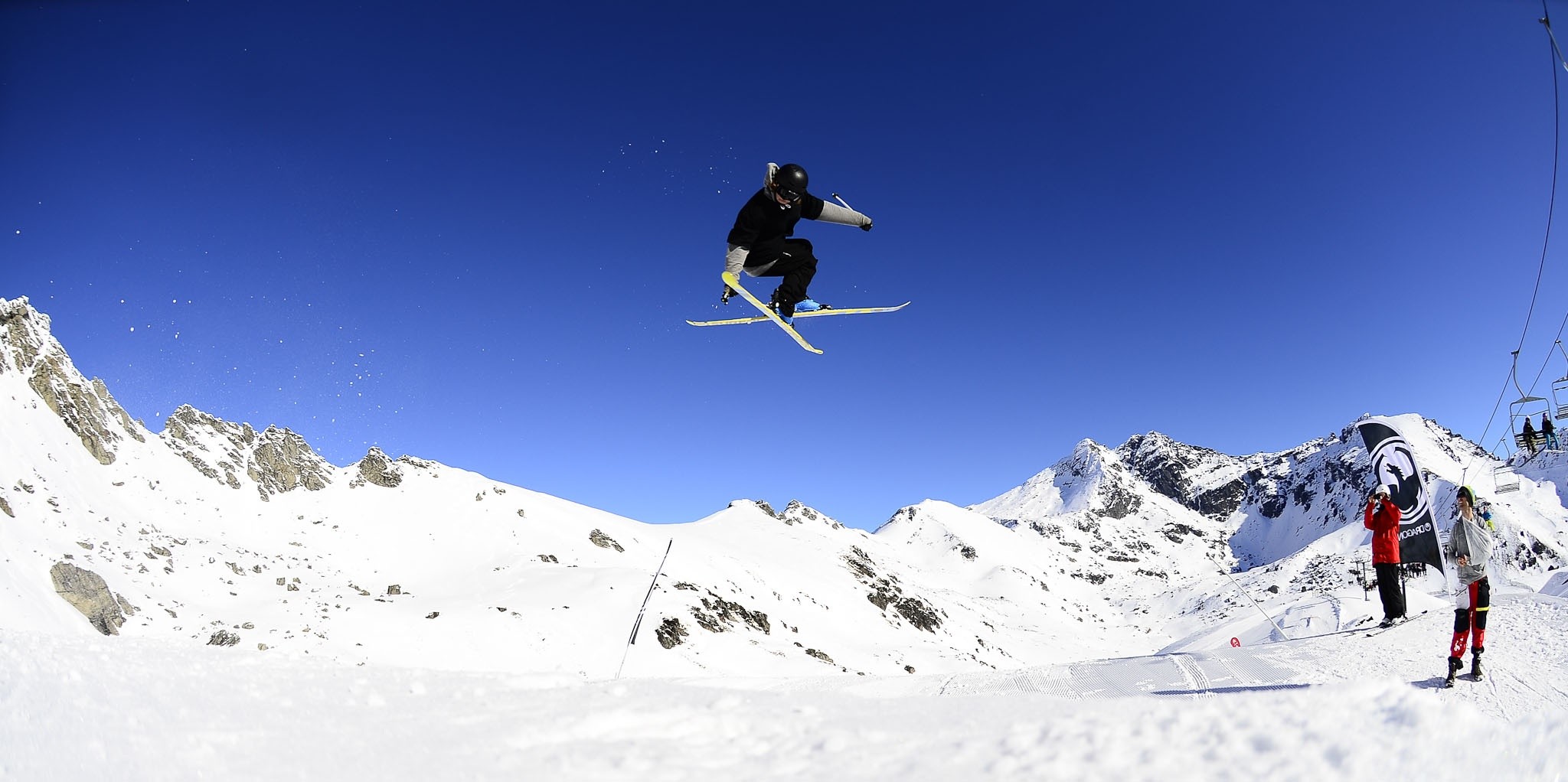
[685,269,911,354]
[1350,610,1428,637]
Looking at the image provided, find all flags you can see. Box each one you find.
[1358,423,1444,575]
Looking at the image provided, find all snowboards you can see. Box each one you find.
[1543,449,1567,454]
[1518,445,1547,467]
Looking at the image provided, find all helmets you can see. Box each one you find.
[1375,484,1390,501]
[774,164,809,195]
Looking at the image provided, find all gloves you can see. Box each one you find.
[859,221,872,232]
[724,284,739,297]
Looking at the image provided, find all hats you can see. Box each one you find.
[1458,485,1475,507]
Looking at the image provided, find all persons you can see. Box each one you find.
[1364,484,1405,627]
[1523,417,1538,453]
[723,161,872,328]
[1446,486,1492,687]
[1542,413,1562,450]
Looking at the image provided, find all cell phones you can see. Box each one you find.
[1373,494,1382,499]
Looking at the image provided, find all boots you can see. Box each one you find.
[1445,663,1457,687]
[1471,658,1483,681]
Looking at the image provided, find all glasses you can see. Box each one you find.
[776,186,799,201]
[1456,492,1465,499]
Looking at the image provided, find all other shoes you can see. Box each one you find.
[1391,615,1406,625]
[1378,618,1391,628]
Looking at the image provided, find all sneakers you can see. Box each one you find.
[775,307,793,325]
[794,299,821,312]
